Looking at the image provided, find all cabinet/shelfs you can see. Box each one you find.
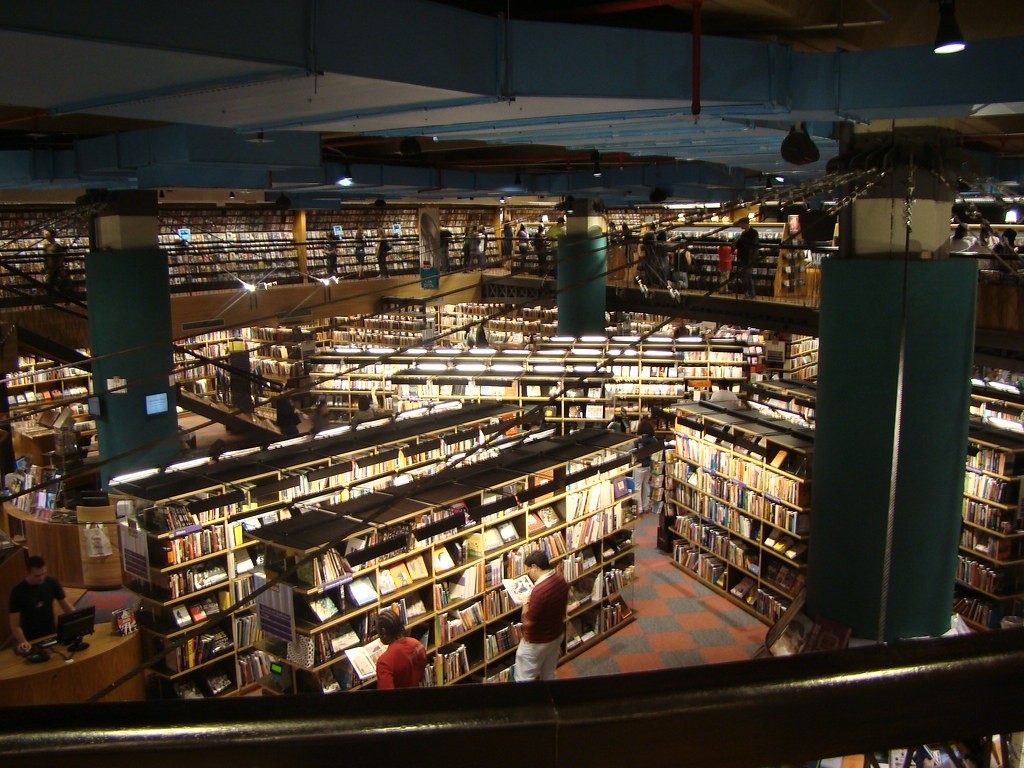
[174,337,237,417]
[232,318,321,421]
[366,206,419,275]
[243,426,642,695]
[667,398,816,637]
[537,332,749,398]
[0,203,228,308]
[760,329,819,382]
[109,400,527,695]
[440,208,502,269]
[330,300,438,350]
[5,347,99,450]
[391,368,616,435]
[751,378,1024,631]
[306,353,688,432]
[433,298,558,346]
[222,205,367,287]
[302,323,331,351]
[634,220,809,299]
[970,394,1024,422]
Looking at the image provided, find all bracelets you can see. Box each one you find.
[523,602,530,605]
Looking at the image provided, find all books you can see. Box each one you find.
[141,302,819,698]
[0,201,830,297]
[5,355,97,512]
[953,441,1020,626]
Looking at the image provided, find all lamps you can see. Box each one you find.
[108,0,1024,568]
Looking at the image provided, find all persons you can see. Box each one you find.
[973,220,1000,249]
[733,218,760,299]
[462,225,488,273]
[546,217,567,281]
[376,226,391,280]
[950,223,971,251]
[275,380,299,430]
[43,230,71,309]
[322,231,338,281]
[375,610,428,690]
[717,235,738,294]
[9,556,77,653]
[634,419,659,514]
[643,221,692,289]
[517,223,531,275]
[503,220,513,270]
[533,224,547,277]
[439,222,453,275]
[355,229,367,279]
[513,550,569,682]
[609,221,632,258]
[990,228,1018,272]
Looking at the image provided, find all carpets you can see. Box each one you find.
[74,588,134,624]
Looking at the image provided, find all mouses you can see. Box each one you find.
[23,647,30,657]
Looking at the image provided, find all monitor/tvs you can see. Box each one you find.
[56,605,95,652]
[145,392,168,414]
[88,396,102,417]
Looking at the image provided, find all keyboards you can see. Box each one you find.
[35,636,57,646]
